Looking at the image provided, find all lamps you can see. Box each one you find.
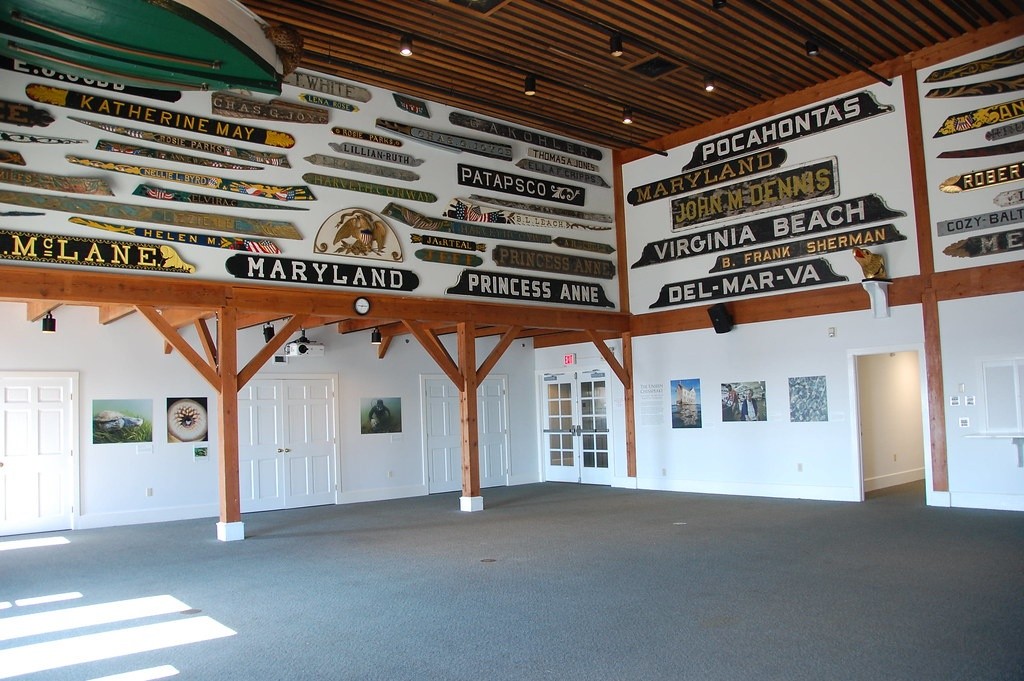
[397,1,820,129]
[371,326,382,345]
[43,310,56,333]
[262,322,275,344]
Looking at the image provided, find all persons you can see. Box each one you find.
[369,399,391,426]
[724,384,763,420]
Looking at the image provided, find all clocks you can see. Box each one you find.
[354,296,370,315]
